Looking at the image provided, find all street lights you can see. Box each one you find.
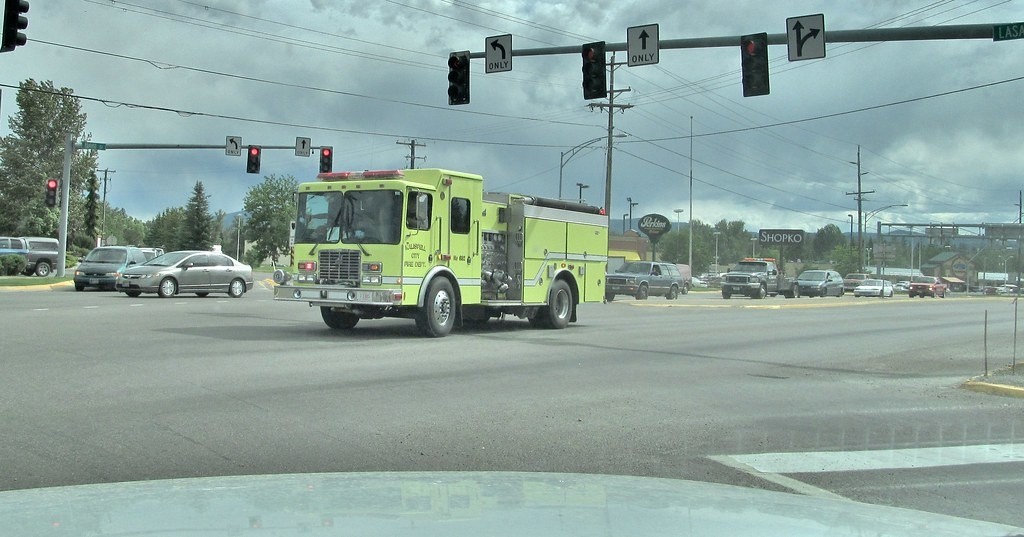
[623,214,629,234]
[559,134,627,199]
[866,247,872,267]
[1005,255,1013,285]
[848,215,853,249]
[627,197,638,229]
[750,237,757,258]
[864,204,909,274]
[577,183,590,203]
[674,209,684,261]
[713,232,721,279]
[236,214,242,261]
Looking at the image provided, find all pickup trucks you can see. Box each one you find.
[908,276,947,298]
[721,258,799,299]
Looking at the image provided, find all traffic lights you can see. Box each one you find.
[45,181,57,207]
[740,32,770,97]
[247,145,261,174]
[320,146,333,173]
[448,50,470,106]
[582,41,607,99]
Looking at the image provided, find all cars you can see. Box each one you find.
[73,247,254,298]
[854,279,893,298]
[985,284,1024,296]
[692,277,708,288]
[798,269,844,297]
[893,280,910,292]
[844,273,872,291]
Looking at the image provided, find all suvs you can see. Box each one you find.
[607,261,692,302]
[0,236,59,277]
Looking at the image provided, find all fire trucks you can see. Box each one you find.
[273,168,609,338]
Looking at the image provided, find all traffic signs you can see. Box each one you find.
[295,137,311,157]
[485,34,514,73]
[225,136,242,156]
[627,23,660,67]
